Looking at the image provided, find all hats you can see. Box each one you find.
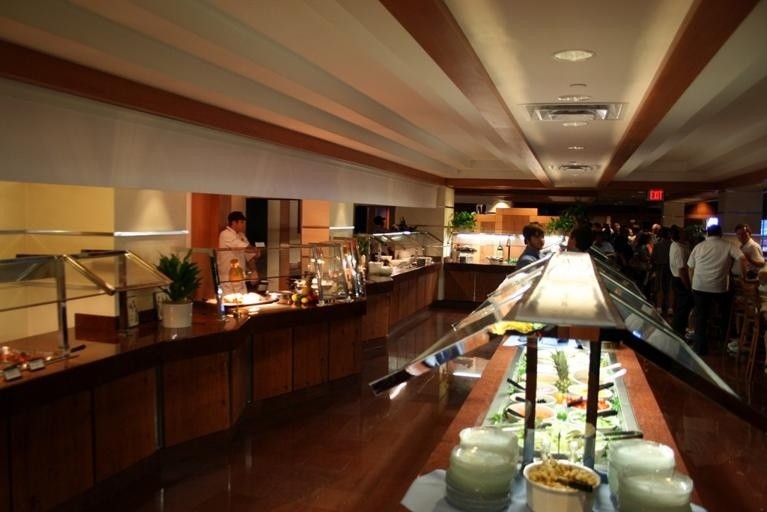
[227,211,250,223]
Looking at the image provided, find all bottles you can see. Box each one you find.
[228,258,248,295]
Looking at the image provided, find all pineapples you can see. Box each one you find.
[551,348,573,394]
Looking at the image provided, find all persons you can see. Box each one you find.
[215,212,261,297]
[531,225,599,341]
[594,220,766,375]
[370,215,387,262]
[516,221,545,270]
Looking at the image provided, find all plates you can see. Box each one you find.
[443,425,519,512]
[606,438,697,512]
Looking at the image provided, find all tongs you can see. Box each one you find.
[566,430,645,445]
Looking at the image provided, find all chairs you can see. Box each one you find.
[724,284,767,406]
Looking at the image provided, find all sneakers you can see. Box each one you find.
[727,337,751,354]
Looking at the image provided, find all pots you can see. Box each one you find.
[368,260,384,274]
[522,460,602,512]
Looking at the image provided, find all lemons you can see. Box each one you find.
[291,287,316,304]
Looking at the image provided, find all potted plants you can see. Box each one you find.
[153,249,203,329]
[546,212,573,235]
[452,210,477,234]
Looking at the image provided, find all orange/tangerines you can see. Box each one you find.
[558,411,567,421]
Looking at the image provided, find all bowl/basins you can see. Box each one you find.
[563,383,620,435]
[378,265,393,277]
[505,373,561,424]
